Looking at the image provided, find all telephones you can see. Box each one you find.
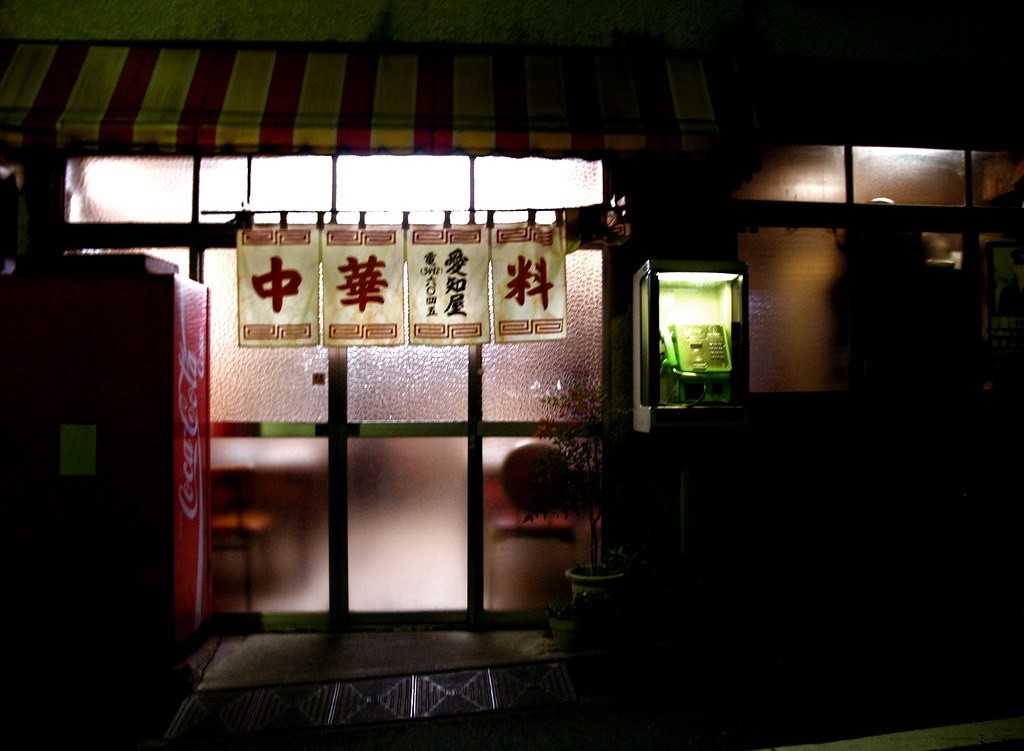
[659,323,732,384]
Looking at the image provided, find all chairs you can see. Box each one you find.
[212,471,277,604]
[488,445,575,541]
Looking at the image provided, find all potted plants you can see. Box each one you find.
[549,347,650,648]
